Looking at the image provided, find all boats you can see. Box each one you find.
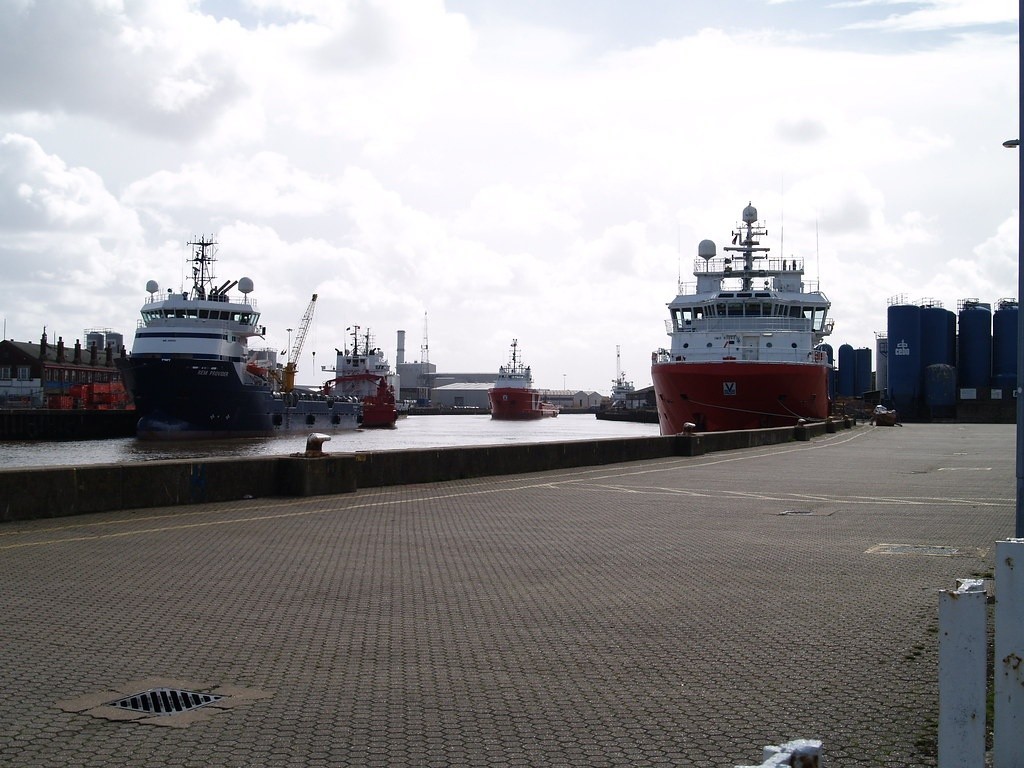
[113,237,366,440]
[651,201,836,438]
[488,337,564,418]
[321,326,400,427]
